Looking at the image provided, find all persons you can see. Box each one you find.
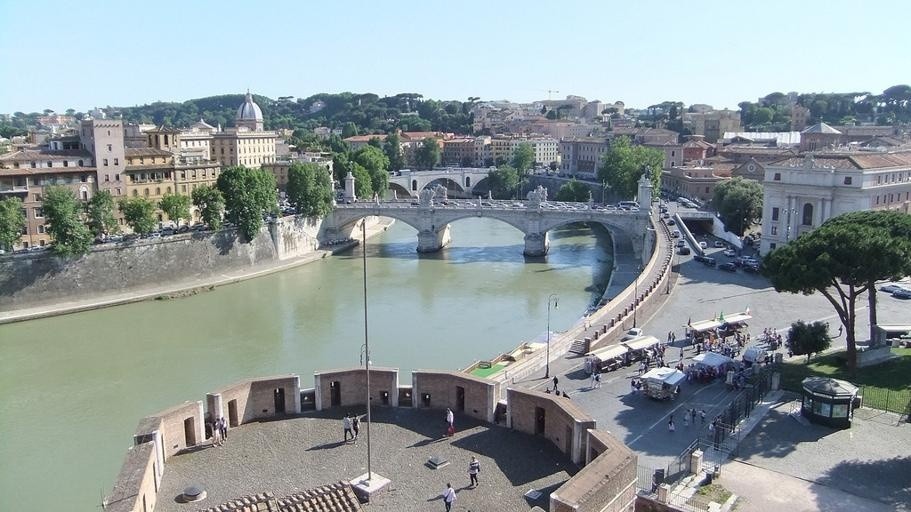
[220,417,228,440]
[446,408,454,426]
[630,327,783,432]
[594,372,601,389]
[838,325,843,336]
[212,417,222,448]
[590,372,595,387]
[343,412,354,442]
[553,376,558,390]
[443,483,457,512]
[467,455,480,486]
[352,413,367,439]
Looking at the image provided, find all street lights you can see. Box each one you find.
[632,262,641,327]
[545,293,558,378]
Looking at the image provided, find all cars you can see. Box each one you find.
[624,328,641,341]
[719,256,760,273]
[724,250,735,257]
[91,232,142,242]
[441,200,524,207]
[894,288,910,299]
[670,195,697,208]
[279,200,298,214]
[882,284,901,291]
[662,208,675,226]
[744,231,761,243]
[714,241,723,248]
[672,230,690,255]
[149,220,235,238]
[699,242,708,249]
[542,201,639,211]
